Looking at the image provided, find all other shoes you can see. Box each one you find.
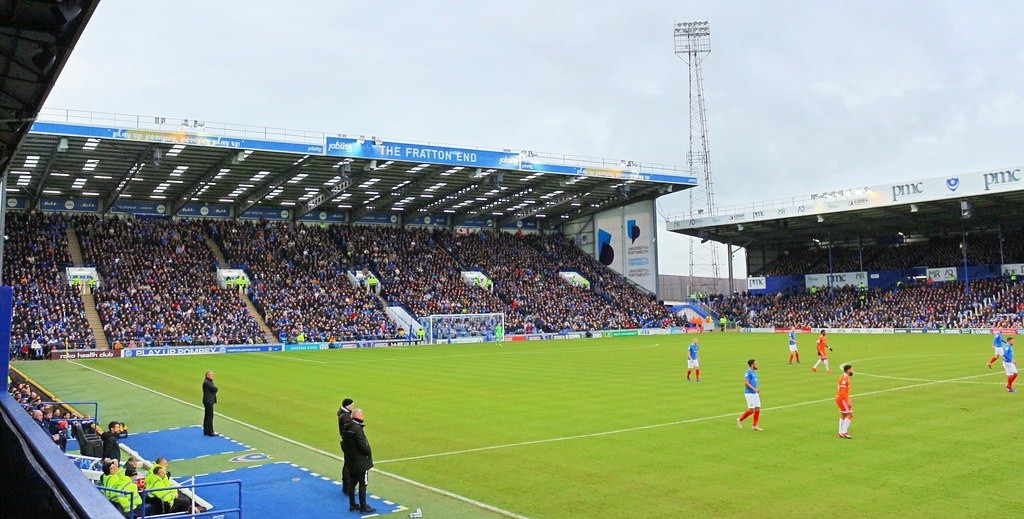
[204,432,219,436]
[788,361,800,365]
[687,377,691,382]
[812,367,818,373]
[1005,385,1017,393]
[826,370,832,374]
[342,490,357,496]
[696,379,701,383]
[988,363,992,369]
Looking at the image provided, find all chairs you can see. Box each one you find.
[72,420,102,457]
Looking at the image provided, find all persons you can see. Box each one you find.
[736,358,764,432]
[986,329,1007,369]
[336,397,358,496]
[1,207,700,361]
[342,408,377,513]
[701,223,1023,333]
[202,370,219,437]
[812,330,834,374]
[685,337,703,382]
[835,365,854,440]
[788,324,802,364]
[1002,337,1019,393]
[6,364,196,518]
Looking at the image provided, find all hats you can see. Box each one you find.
[102,462,114,475]
[125,468,138,476]
[342,398,354,407]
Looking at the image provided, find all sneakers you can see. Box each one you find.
[837,433,852,439]
[359,505,376,513]
[737,417,743,430]
[349,504,359,511]
[752,426,763,431]
